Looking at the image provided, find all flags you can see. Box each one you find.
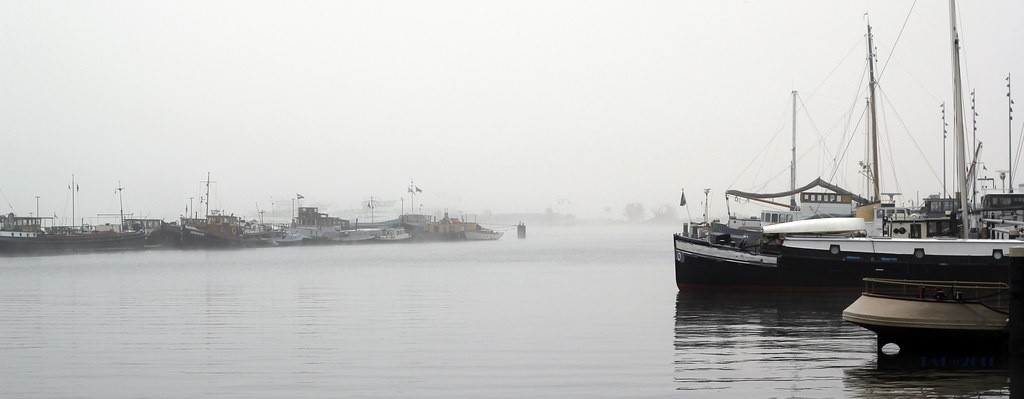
[367,201,371,209]
[297,193,304,200]
[408,187,415,195]
[77,184,79,192]
[68,185,71,190]
[416,186,422,193]
[679,192,687,206]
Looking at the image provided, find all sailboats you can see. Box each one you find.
[0,175,502,256]
[674,27,902,305]
[780,0,1024,310]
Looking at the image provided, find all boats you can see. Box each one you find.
[842,277,1012,373]
